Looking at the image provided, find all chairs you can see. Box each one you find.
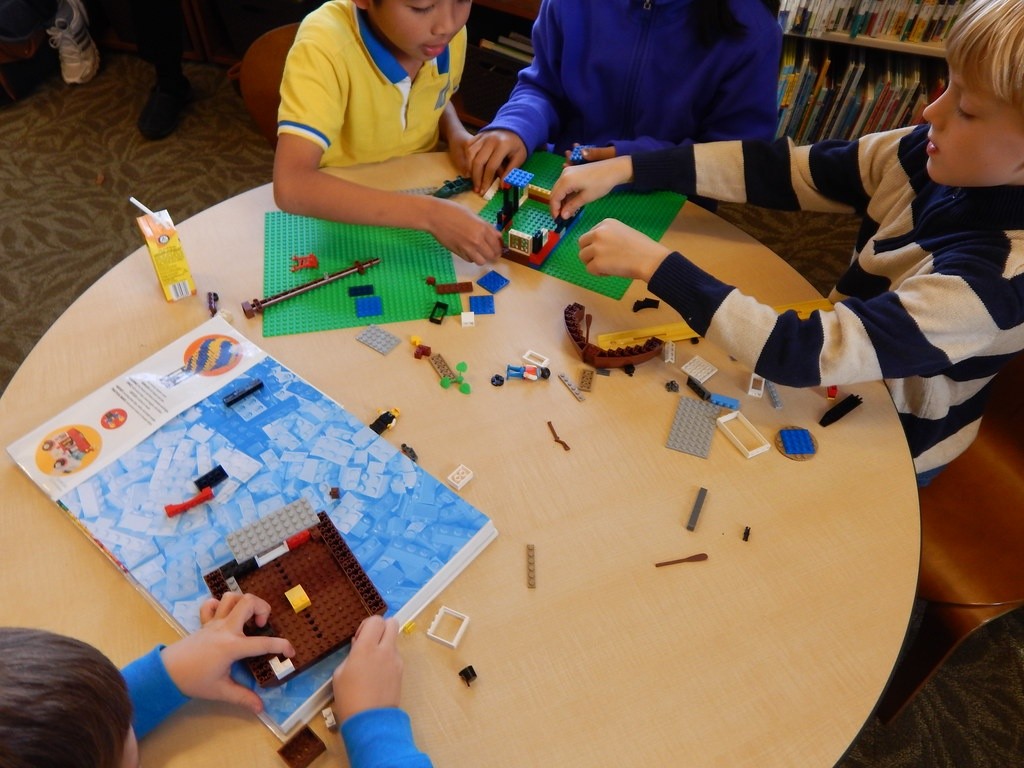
[876,344,1024,725]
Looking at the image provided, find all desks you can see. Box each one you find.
[0,148,920,768]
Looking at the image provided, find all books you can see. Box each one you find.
[770,0,970,141]
[478,31,542,64]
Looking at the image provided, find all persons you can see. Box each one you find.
[1,0,100,83]
[549,0,1024,490]
[132,0,196,138]
[463,0,784,198]
[273,0,506,267]
[0,591,433,768]
